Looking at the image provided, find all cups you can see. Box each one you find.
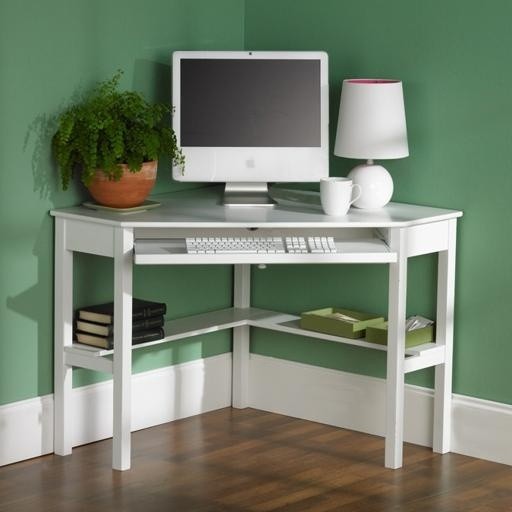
[320,176,362,217]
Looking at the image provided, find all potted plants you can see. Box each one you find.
[51,69,186,212]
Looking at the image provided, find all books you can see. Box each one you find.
[72,297,167,351]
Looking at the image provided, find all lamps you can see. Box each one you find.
[332,78,409,209]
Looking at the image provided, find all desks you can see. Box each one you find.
[47,185,462,472]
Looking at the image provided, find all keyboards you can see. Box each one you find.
[185,237,338,254]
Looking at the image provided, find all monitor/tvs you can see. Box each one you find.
[171,49,330,208]
